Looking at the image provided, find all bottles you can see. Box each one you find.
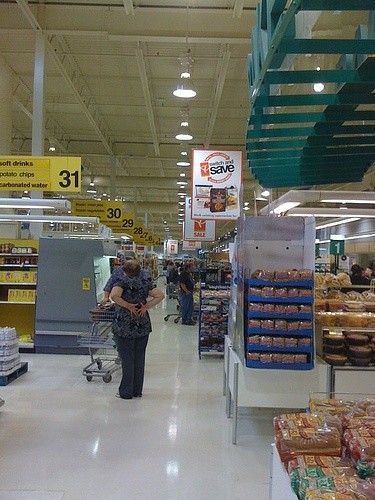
[0,326,21,377]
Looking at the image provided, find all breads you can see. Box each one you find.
[272,397,375,500]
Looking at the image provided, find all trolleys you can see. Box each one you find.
[165,282,200,324]
[77,300,123,383]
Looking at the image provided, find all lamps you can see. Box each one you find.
[172,33,197,225]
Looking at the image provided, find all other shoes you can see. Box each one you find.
[182,320,194,326]
[190,319,196,324]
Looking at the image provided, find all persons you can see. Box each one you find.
[109,261,166,399]
[345,263,375,285]
[137,253,207,300]
[100,256,147,364]
[177,261,198,326]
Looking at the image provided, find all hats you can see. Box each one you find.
[125,251,136,260]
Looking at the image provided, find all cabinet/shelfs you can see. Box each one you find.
[198,251,233,360]
[224,214,375,500]
[0,238,40,353]
